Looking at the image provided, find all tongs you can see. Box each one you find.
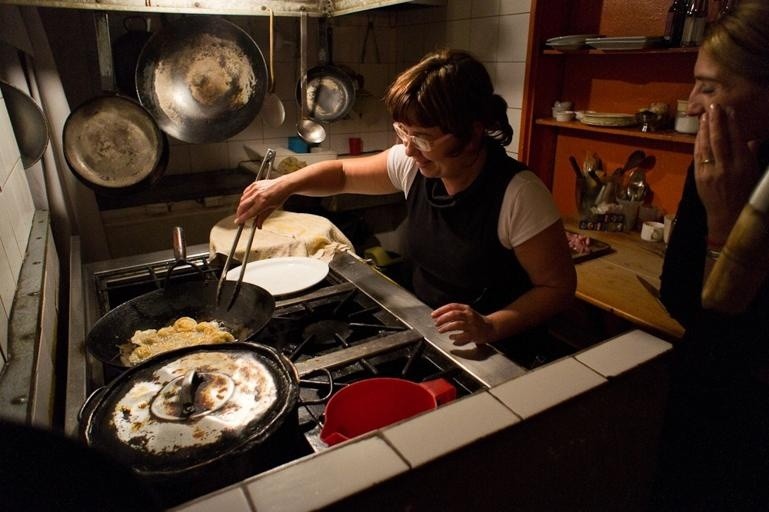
[215,150,276,312]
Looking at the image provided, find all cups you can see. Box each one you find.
[552,105,570,118]
[675,99,701,134]
[556,110,575,121]
[347,137,363,155]
[664,213,676,245]
[639,222,665,243]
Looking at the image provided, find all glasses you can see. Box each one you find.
[395,122,452,152]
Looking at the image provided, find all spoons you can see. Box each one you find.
[293,12,327,143]
[624,148,648,171]
[261,9,287,131]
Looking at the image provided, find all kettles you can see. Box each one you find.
[663,0,709,46]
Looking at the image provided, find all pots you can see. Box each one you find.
[60,4,163,195]
[80,224,276,369]
[296,14,359,124]
[77,337,333,477]
[133,15,271,147]
[0,82,51,168]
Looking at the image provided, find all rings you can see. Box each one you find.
[702,159,715,165]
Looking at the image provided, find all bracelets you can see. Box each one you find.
[704,246,721,260]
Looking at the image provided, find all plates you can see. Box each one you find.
[579,113,642,127]
[546,34,588,51]
[585,34,650,51]
[224,256,329,297]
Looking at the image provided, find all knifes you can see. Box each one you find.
[634,272,676,320]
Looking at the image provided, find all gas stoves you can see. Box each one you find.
[90,249,491,492]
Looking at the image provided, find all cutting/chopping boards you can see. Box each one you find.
[556,229,612,260]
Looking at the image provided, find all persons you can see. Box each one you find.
[658,3,769,512]
[234,50,578,369]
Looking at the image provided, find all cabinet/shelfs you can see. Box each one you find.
[522,0,749,339]
